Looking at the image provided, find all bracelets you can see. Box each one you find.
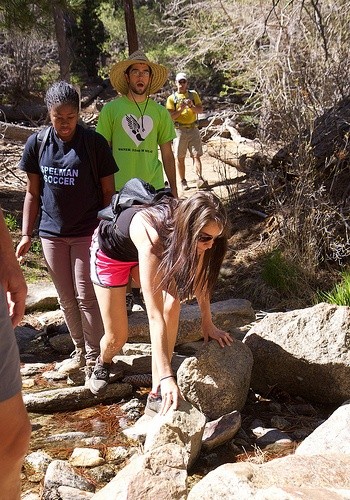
[22,234,30,237]
[160,375,174,380]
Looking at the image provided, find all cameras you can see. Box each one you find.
[183,99,190,104]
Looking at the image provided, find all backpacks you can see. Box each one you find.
[97,177,175,244]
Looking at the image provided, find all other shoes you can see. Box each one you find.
[56,347,87,374]
[197,179,208,189]
[84,358,96,375]
[125,292,147,316]
[181,181,189,190]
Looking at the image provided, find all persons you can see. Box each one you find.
[15,81,117,373]
[95,51,178,202]
[1,210,31,498]
[89,189,234,413]
[166,72,210,189]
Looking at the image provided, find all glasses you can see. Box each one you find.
[198,234,223,242]
[178,81,186,83]
[128,71,150,76]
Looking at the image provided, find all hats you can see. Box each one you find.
[176,73,187,82]
[109,50,168,96]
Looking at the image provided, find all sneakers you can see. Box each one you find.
[88,354,114,396]
[143,392,170,415]
